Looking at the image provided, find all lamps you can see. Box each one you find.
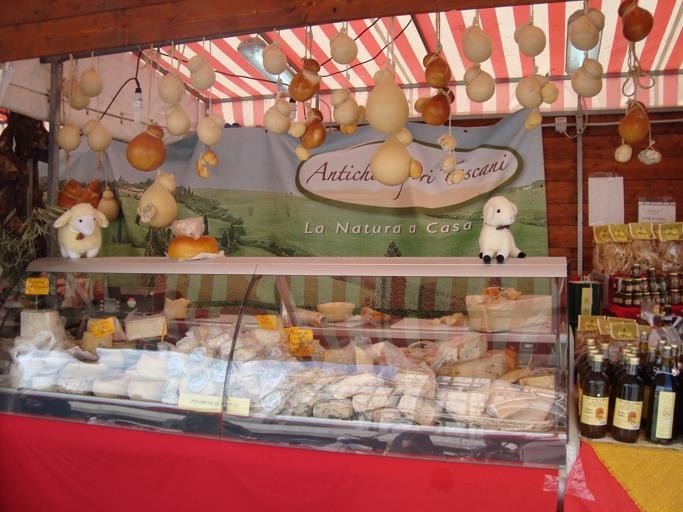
[131,73,141,124]
[564,10,603,77]
[237,37,317,103]
[286,92,296,123]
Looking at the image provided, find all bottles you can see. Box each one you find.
[94,280,103,312]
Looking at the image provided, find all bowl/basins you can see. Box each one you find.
[317,302,354,321]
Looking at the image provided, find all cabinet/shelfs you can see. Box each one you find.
[0,256,580,511]
[586,268,682,324]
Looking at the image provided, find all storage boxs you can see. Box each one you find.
[466,286,553,335]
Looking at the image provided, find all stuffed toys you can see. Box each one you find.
[54,203,109,259]
[479,195,526,264]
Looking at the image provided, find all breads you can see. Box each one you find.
[293,338,556,430]
[168,237,219,259]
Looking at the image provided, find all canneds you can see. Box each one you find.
[612,262,683,315]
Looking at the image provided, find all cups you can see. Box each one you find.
[575,338,681,445]
